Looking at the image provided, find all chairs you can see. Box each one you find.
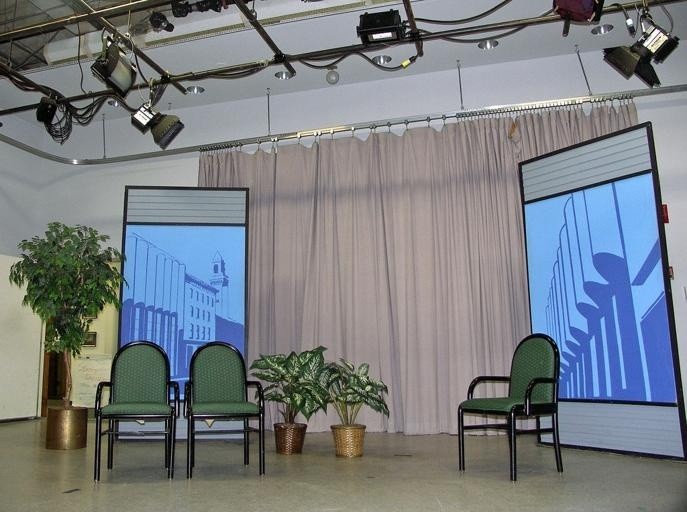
[181,338,265,478]
[95,339,181,482]
[455,333,563,481]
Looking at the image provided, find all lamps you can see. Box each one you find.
[87,0,240,150]
[600,21,679,91]
[548,1,609,29]
[351,9,409,47]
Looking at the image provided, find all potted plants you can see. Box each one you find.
[318,356,389,458]
[249,344,336,455]
[6,217,128,451]
